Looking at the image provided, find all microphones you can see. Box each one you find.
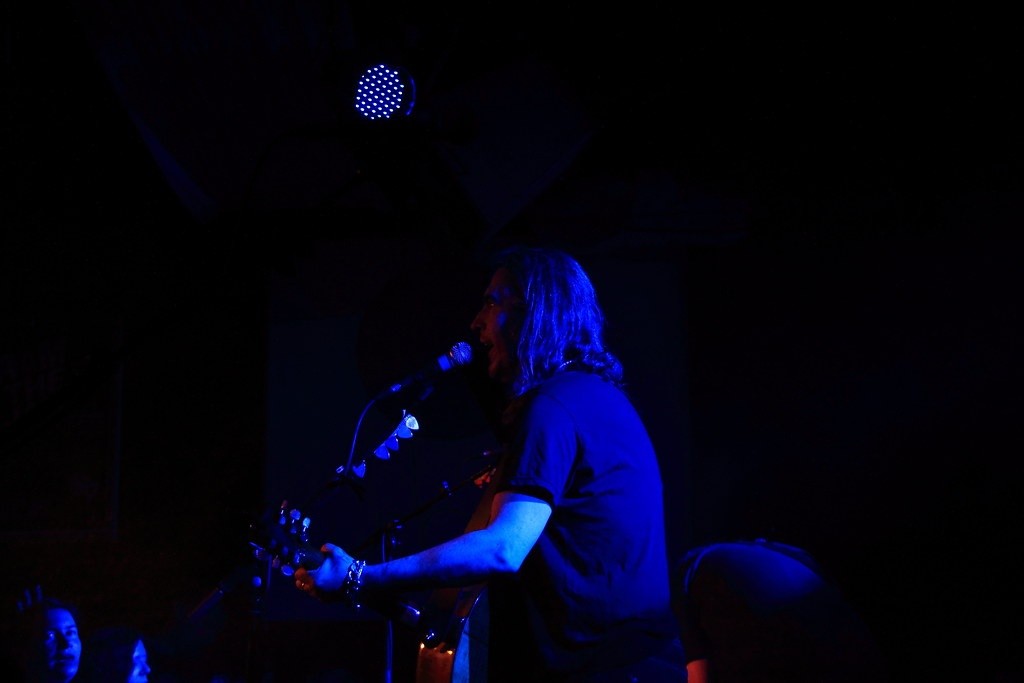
[381,341,475,398]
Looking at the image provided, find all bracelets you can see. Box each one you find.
[343,559,367,607]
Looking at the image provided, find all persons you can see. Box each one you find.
[21,598,83,683]
[679,537,875,683]
[276,250,688,682]
[95,628,154,683]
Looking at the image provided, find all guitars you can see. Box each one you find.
[267,500,491,683]
